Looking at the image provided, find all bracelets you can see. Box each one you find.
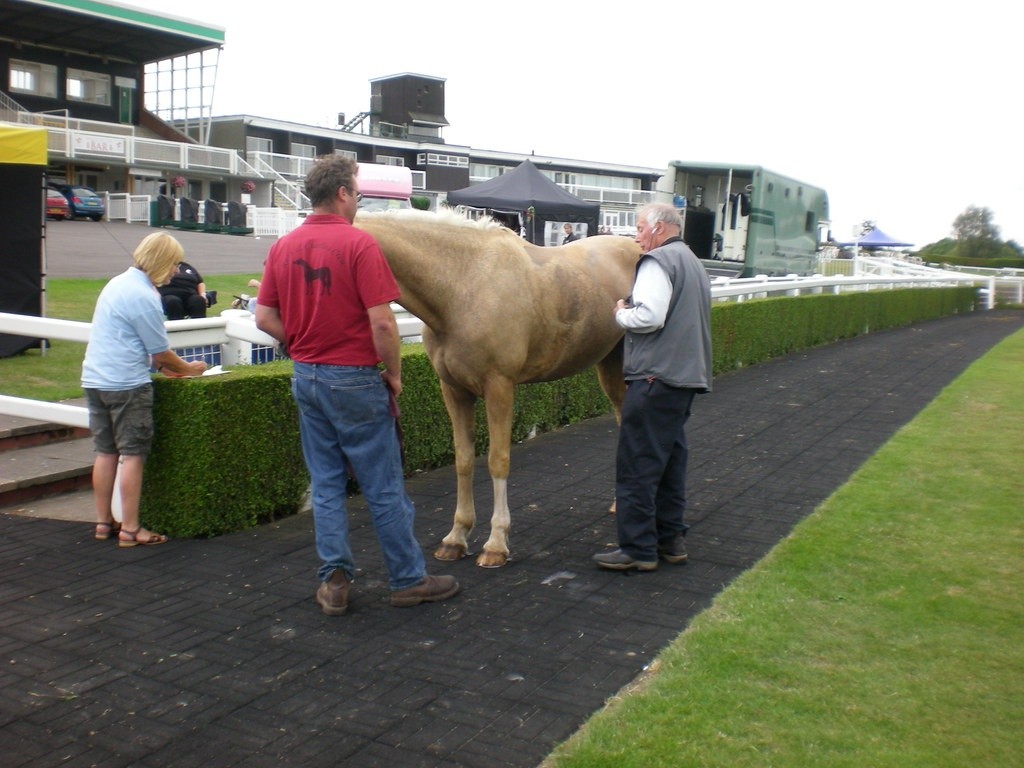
[158,364,163,372]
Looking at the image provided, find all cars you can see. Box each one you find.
[44,185,68,221]
[59,185,105,222]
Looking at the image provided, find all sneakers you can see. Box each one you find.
[390,574,459,607]
[592,549,658,570]
[657,531,687,563]
[317,568,349,615]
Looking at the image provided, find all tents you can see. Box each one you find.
[448,158,600,246]
[1,124,52,361]
[839,227,912,247]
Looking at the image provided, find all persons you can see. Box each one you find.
[79,230,210,547]
[562,225,577,245]
[155,261,209,323]
[254,149,462,616]
[237,260,267,312]
[590,203,714,571]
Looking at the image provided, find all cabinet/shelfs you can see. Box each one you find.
[710,201,750,261]
[684,207,714,258]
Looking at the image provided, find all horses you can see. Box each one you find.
[345,205,646,569]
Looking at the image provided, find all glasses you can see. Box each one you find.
[173,262,182,268]
[345,185,363,203]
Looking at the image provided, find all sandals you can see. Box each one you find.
[118,526,168,547]
[95,519,122,539]
